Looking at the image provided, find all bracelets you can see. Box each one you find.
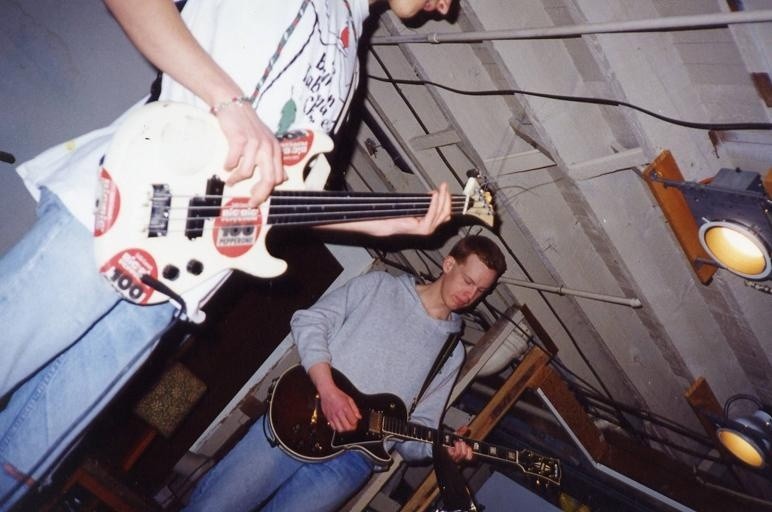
[209,92,254,118]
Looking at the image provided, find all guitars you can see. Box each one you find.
[92,101,497,323]
[265,364,563,495]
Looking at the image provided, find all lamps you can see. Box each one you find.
[650,165,772,300]
[699,389,771,481]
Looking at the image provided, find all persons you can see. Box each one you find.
[1,0,462,511]
[179,233,511,510]
